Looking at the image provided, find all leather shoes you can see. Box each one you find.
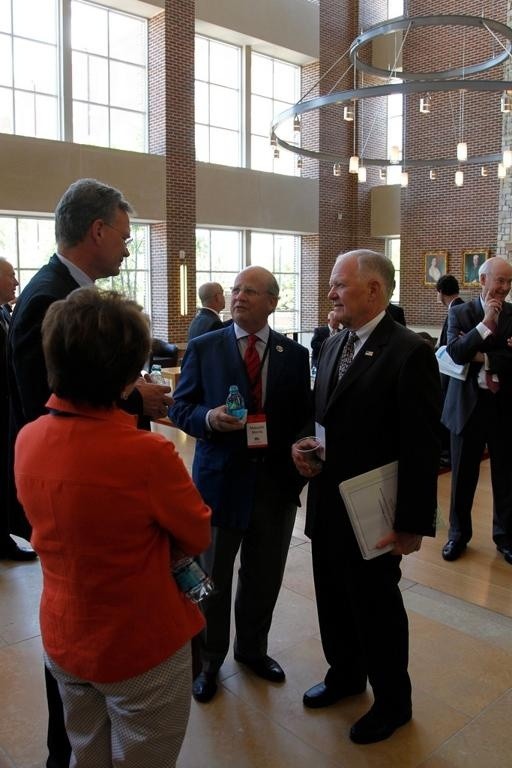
[349,706,412,744]
[243,655,285,682]
[496,546,512,564]
[191,674,217,703]
[442,540,467,560]
[303,681,366,709]
[9,545,39,561]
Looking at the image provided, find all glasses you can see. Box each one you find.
[103,222,133,245]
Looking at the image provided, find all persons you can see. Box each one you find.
[436,273,465,464]
[0,256,38,561]
[186,283,228,341]
[14,282,214,765]
[469,254,485,283]
[170,265,312,701]
[428,258,441,281]
[389,308,407,326]
[442,257,512,561]
[311,310,344,371]
[296,249,438,743]
[6,179,175,768]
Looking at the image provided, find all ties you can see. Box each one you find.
[486,321,500,394]
[243,335,262,412]
[334,331,359,388]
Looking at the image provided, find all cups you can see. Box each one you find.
[293,437,322,477]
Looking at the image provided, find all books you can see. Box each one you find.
[338,459,424,561]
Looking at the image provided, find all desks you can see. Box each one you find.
[278,329,314,342]
[159,367,181,389]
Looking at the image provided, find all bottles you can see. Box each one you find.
[226,384,245,420]
[169,546,215,605]
[148,365,164,385]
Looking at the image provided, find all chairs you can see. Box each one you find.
[143,339,178,374]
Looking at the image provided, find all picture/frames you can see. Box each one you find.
[423,251,449,286]
[460,248,491,288]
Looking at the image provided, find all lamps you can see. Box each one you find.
[270,0,512,187]
